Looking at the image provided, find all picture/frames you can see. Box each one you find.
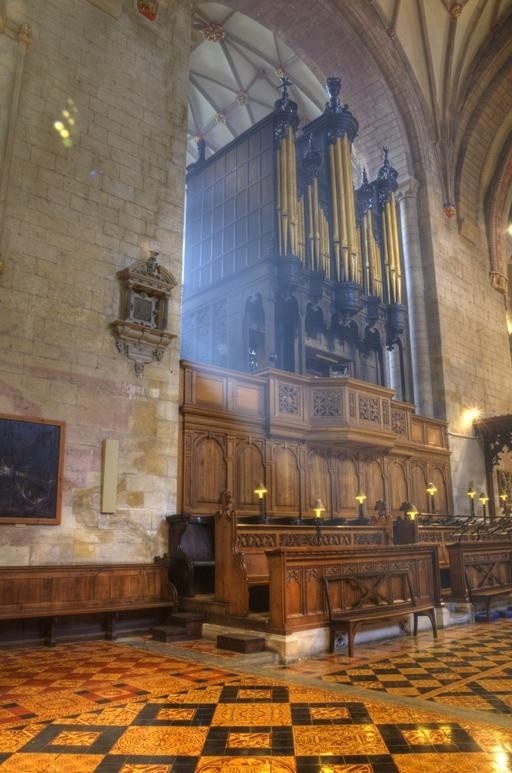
[1,412,67,526]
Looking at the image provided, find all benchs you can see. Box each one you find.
[320,558,511,657]
